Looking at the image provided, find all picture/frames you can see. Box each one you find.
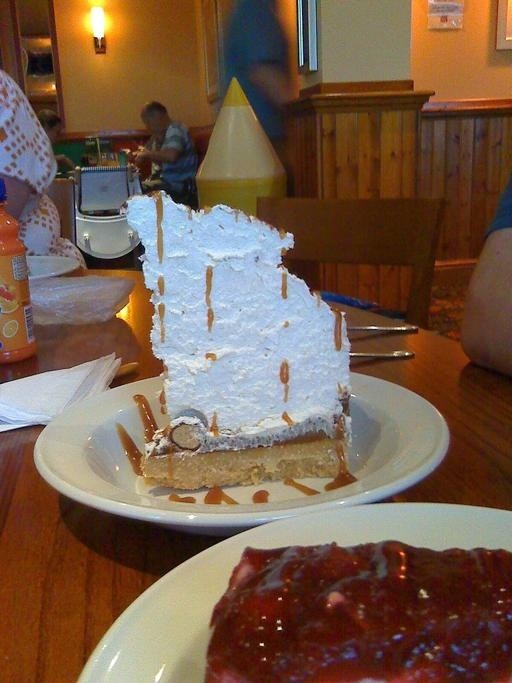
[496,0,512,50]
[296,0,317,75]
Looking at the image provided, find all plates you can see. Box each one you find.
[69,500,510,681]
[32,371,452,540]
[24,254,81,281]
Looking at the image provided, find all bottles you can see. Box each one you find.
[0,176,38,365]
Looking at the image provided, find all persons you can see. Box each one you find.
[453,167,512,382]
[128,95,199,207]
[210,3,302,198]
[0,64,88,273]
[36,106,79,174]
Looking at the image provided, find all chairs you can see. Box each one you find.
[257,197,446,328]
[48,179,76,245]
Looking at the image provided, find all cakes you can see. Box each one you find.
[203,541,512,683]
[112,192,357,493]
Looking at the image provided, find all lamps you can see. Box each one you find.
[91,8,106,54]
